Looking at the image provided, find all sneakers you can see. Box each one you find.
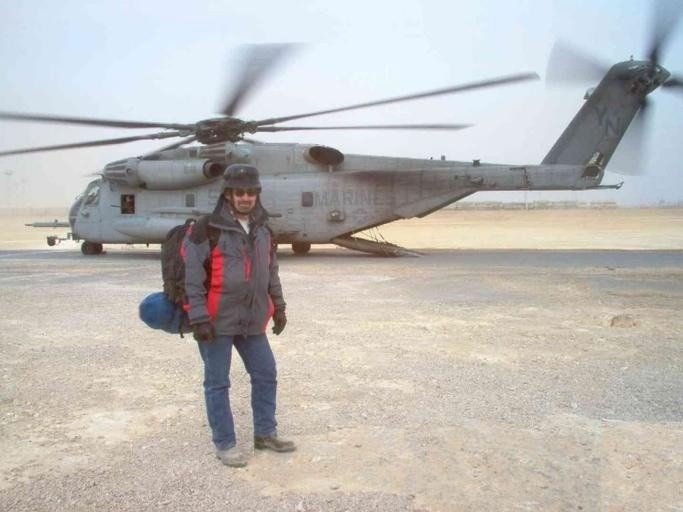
[254,435,296,453]
[216,447,248,468]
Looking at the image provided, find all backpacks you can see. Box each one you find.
[161,218,216,306]
[139,292,193,334]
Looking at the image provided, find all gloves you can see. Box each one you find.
[193,322,217,343]
[272,307,287,336]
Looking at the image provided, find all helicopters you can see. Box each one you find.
[1,0,683,258]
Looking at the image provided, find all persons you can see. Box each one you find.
[184,161,298,470]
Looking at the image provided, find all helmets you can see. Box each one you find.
[223,163,262,188]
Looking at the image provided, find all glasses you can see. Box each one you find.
[233,190,259,196]
